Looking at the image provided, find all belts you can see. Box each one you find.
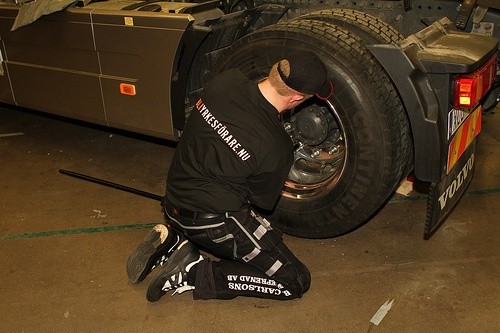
[162,196,224,218]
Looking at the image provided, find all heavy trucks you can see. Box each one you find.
[0,0,499,241]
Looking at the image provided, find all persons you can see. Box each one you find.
[126,51,334,302]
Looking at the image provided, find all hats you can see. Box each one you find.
[277,50,334,100]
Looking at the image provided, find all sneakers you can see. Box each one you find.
[146,239,206,302]
[126,223,180,284]
